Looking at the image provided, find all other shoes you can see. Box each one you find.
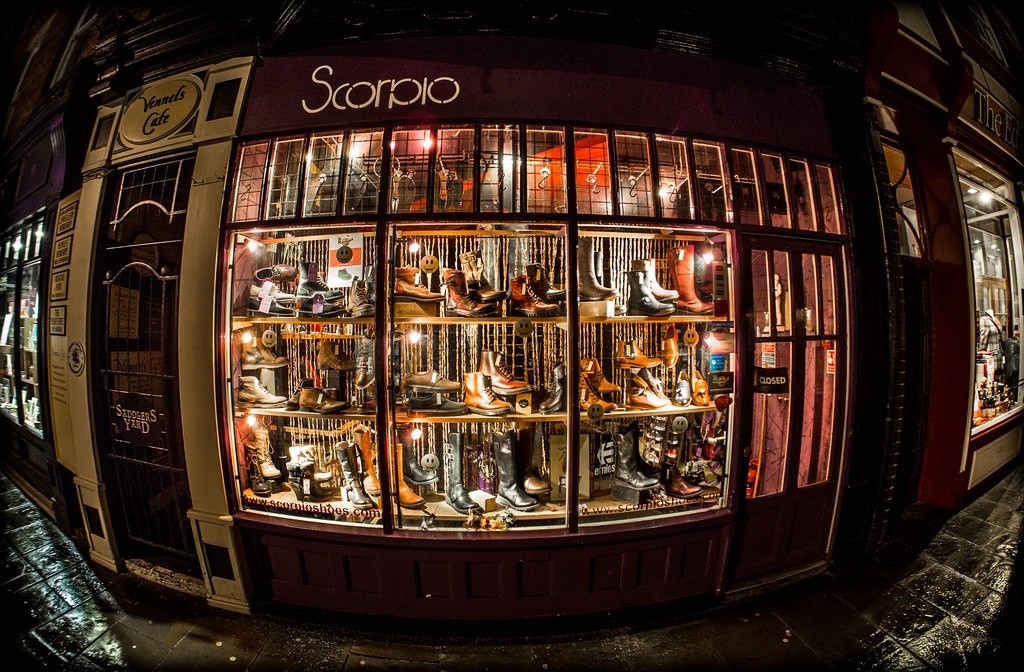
[317,340,357,371]
[625,369,673,411]
[246,265,296,318]
[406,369,462,415]
[284,377,350,413]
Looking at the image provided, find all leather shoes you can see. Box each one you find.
[675,370,709,407]
[356,397,377,414]
[615,340,661,369]
[355,334,376,389]
[660,462,703,498]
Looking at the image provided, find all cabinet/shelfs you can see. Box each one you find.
[230,220,739,532]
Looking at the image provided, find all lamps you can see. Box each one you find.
[541,163,551,176]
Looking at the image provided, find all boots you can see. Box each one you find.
[538,362,565,415]
[666,245,714,315]
[395,268,445,302]
[579,358,617,412]
[295,262,345,317]
[463,350,531,416]
[240,421,550,513]
[628,258,679,315]
[510,264,566,316]
[578,237,626,316]
[444,250,506,317]
[618,420,660,477]
[612,428,659,490]
[345,272,377,317]
[238,334,287,408]
